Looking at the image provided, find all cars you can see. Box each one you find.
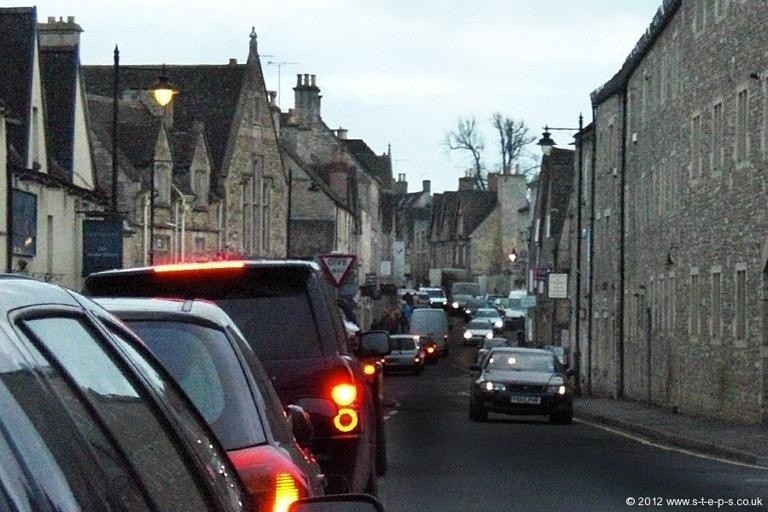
[1,259,573,512]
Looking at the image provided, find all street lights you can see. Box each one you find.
[536,112,583,393]
[110,42,180,268]
